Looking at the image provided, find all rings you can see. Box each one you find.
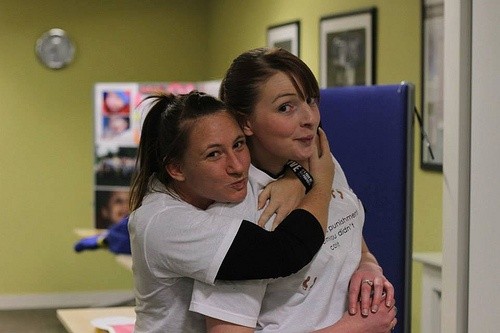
[362,280,373,287]
[391,320,394,330]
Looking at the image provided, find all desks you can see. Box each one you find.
[55,306,137,333]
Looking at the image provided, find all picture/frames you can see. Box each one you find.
[420,0,444,172]
[267,19,300,58]
[319,6,377,89]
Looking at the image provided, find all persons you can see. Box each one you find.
[190,45,397,333]
[103,92,129,139]
[96,156,129,186]
[128,90,334,333]
[104,192,133,223]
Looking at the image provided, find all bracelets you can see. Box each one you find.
[284,159,313,195]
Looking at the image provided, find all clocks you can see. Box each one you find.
[35,28,76,69]
